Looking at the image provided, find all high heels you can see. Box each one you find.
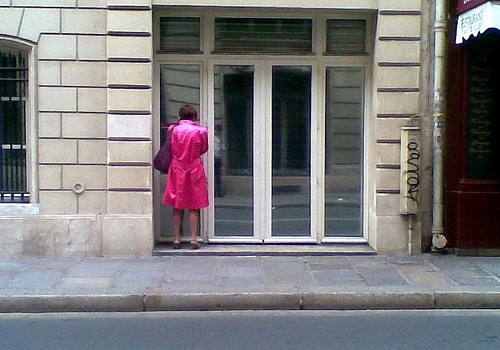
[172,240,181,252]
[189,241,202,249]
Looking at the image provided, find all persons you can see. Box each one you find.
[214,123,226,197]
[162,105,209,249]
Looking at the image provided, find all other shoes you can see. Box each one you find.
[214,192,225,198]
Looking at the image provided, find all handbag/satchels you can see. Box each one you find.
[153,125,177,174]
